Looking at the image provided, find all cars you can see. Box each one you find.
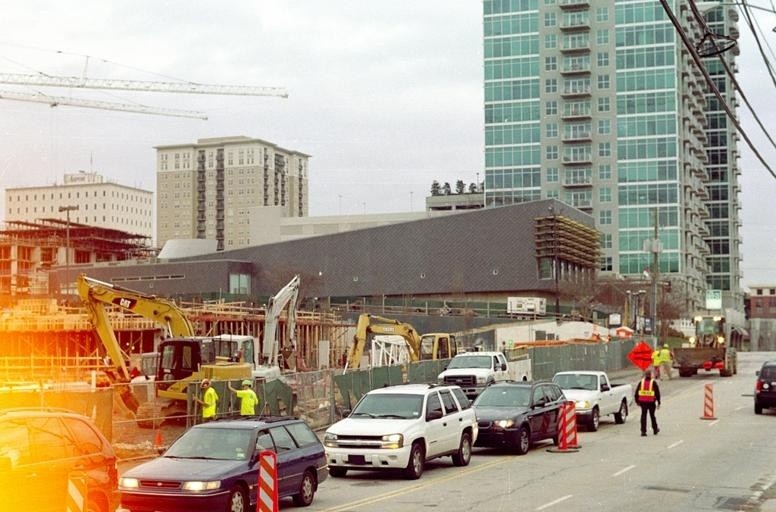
[751,361,776,414]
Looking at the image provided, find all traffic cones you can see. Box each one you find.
[154,428,163,449]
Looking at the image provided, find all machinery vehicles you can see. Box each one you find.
[672,315,741,378]
[65,266,315,420]
[335,309,469,386]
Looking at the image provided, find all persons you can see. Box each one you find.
[192,378,258,424]
[635,342,675,436]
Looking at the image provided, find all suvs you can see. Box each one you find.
[0,405,123,512]
[320,382,479,480]
[117,412,330,510]
[472,378,567,455]
[438,349,509,399]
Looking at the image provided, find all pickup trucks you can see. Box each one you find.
[550,370,634,431]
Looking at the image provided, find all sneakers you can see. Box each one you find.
[641,432,647,436]
[654,428,660,434]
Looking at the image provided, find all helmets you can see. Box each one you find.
[664,344,669,348]
[200,379,210,388]
[241,380,253,388]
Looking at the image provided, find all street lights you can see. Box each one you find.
[548,207,565,322]
[626,288,646,333]
[57,204,79,315]
